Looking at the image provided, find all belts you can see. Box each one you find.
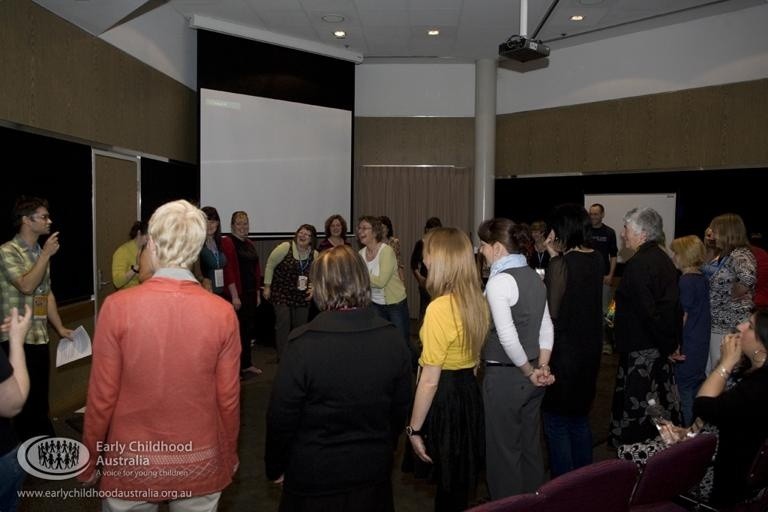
[487,358,539,367]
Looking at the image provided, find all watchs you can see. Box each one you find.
[406,425,422,437]
[130,264,139,274]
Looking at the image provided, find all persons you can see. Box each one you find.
[670,234,711,429]
[75,196,242,512]
[541,209,605,480]
[262,242,413,512]
[304,213,352,323]
[537,363,549,369]
[262,224,320,363]
[691,302,768,511]
[700,226,729,280]
[379,215,407,291]
[476,216,557,502]
[220,210,263,383]
[410,217,442,354]
[525,366,536,379]
[355,215,420,370]
[703,213,758,389]
[0,301,35,510]
[745,239,767,312]
[110,220,150,292]
[0,193,74,439]
[581,203,619,329]
[609,205,686,452]
[404,222,492,512]
[528,221,551,268]
[193,206,228,296]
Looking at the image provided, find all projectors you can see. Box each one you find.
[498,32,552,65]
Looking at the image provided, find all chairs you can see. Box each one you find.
[466,493,548,511]
[628,434,717,512]
[538,458,640,511]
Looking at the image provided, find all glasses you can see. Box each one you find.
[41,215,48,219]
[298,232,312,238]
[356,226,372,231]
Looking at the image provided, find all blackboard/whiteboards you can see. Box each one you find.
[0,126,98,312]
[584,193,677,264]
[137,156,201,222]
[492,167,763,253]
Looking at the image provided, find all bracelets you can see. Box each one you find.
[713,363,731,381]
